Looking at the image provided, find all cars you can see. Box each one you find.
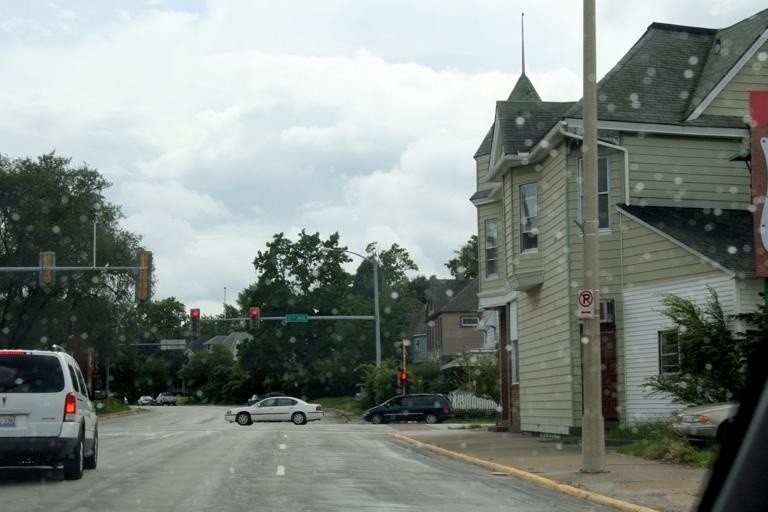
[224,395,324,426]
[1,344,98,480]
[362,393,455,424]
[137,395,156,406]
[670,402,740,449]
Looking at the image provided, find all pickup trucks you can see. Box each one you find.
[156,392,176,406]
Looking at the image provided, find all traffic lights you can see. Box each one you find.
[401,372,408,388]
[249,307,261,330]
[191,308,201,340]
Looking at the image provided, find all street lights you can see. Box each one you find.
[320,246,382,369]
